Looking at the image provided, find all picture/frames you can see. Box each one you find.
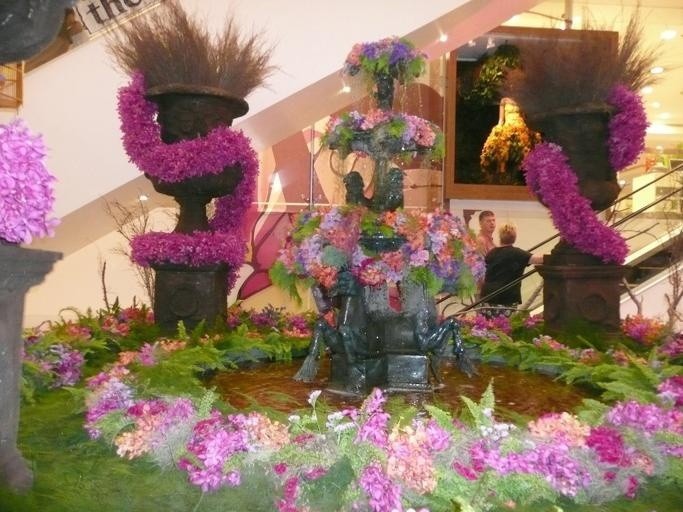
[444,25,618,202]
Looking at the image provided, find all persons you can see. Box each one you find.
[483,98,540,173]
[472,210,496,317]
[481,224,543,315]
